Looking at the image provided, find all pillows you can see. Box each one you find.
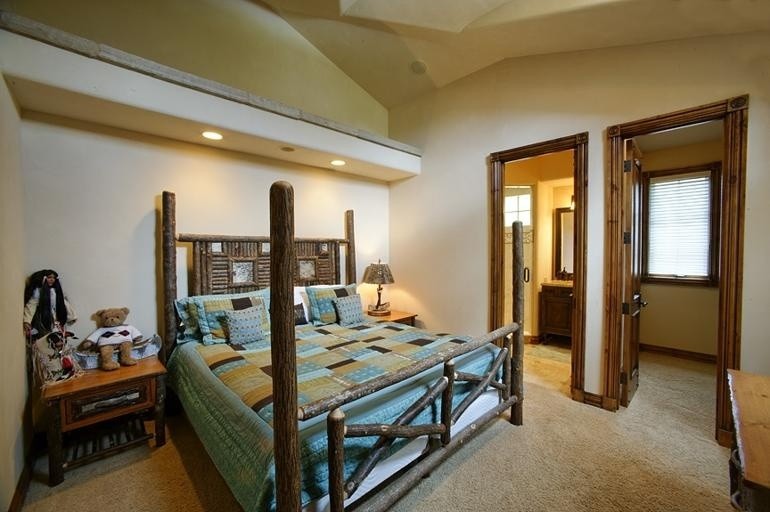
[173,283,364,345]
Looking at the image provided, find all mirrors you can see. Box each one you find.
[555,207,574,280]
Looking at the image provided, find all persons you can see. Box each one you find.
[21,269,78,337]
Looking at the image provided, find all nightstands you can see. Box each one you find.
[41,356,168,488]
[364,308,419,330]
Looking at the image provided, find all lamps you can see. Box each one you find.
[362,259,394,316]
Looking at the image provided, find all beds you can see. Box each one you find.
[160,179,526,511]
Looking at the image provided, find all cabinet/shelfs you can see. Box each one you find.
[539,280,574,345]
[726,368,769,512]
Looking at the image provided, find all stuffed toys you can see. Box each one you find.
[83,307,142,370]
